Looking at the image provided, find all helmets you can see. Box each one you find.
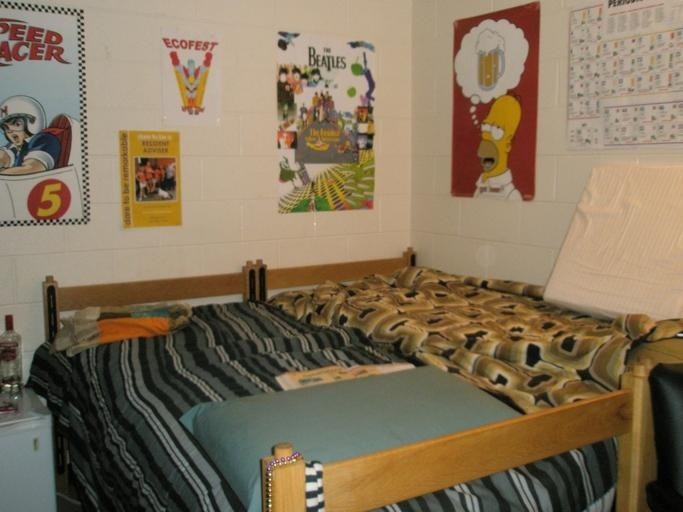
[0,94,46,144]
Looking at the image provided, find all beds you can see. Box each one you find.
[41,247,683,511]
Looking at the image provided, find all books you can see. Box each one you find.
[276,362,417,391]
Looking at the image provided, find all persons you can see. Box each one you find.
[135,159,176,202]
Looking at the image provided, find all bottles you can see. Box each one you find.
[1,314,22,418]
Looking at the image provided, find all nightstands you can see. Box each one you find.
[0,388,56,511]
